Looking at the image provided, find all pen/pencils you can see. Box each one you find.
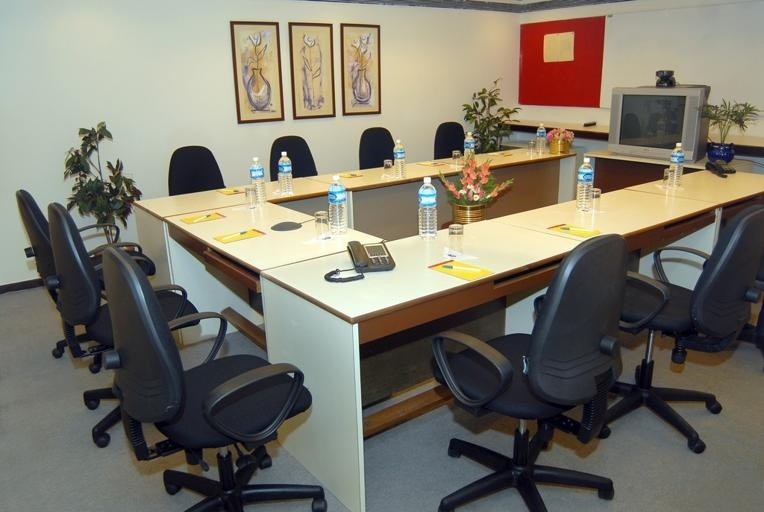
[560,228,593,230]
[193,214,211,223]
[443,265,480,271]
[223,231,247,239]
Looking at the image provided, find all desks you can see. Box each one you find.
[133,148,764,512]
[164,202,388,346]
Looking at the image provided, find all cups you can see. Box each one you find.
[447,223,465,258]
[245,185,257,210]
[452,150,461,161]
[527,139,535,155]
[313,211,328,238]
[662,168,676,189]
[589,187,602,212]
[383,159,393,175]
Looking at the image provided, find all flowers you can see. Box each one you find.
[438,151,514,205]
[547,127,575,141]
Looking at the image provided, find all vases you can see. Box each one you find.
[549,141,570,154]
[452,203,487,225]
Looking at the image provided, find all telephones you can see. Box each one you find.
[347,241,396,273]
[706,159,736,173]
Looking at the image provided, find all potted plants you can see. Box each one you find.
[694,98,764,164]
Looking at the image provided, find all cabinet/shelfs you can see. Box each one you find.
[583,151,752,194]
[499,120,609,151]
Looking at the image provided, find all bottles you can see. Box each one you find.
[536,123,546,155]
[417,175,438,240]
[669,142,684,187]
[278,151,293,195]
[248,157,266,208]
[327,175,347,236]
[576,156,594,212]
[392,138,407,178]
[463,131,476,161]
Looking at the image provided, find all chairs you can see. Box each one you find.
[431,233,627,512]
[270,135,318,182]
[359,127,395,170]
[48,203,200,447]
[102,246,328,512]
[16,190,156,373]
[168,146,226,196]
[434,122,465,160]
[598,203,764,454]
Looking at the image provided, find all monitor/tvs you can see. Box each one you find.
[608,84,713,164]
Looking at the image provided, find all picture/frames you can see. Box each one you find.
[230,21,381,124]
